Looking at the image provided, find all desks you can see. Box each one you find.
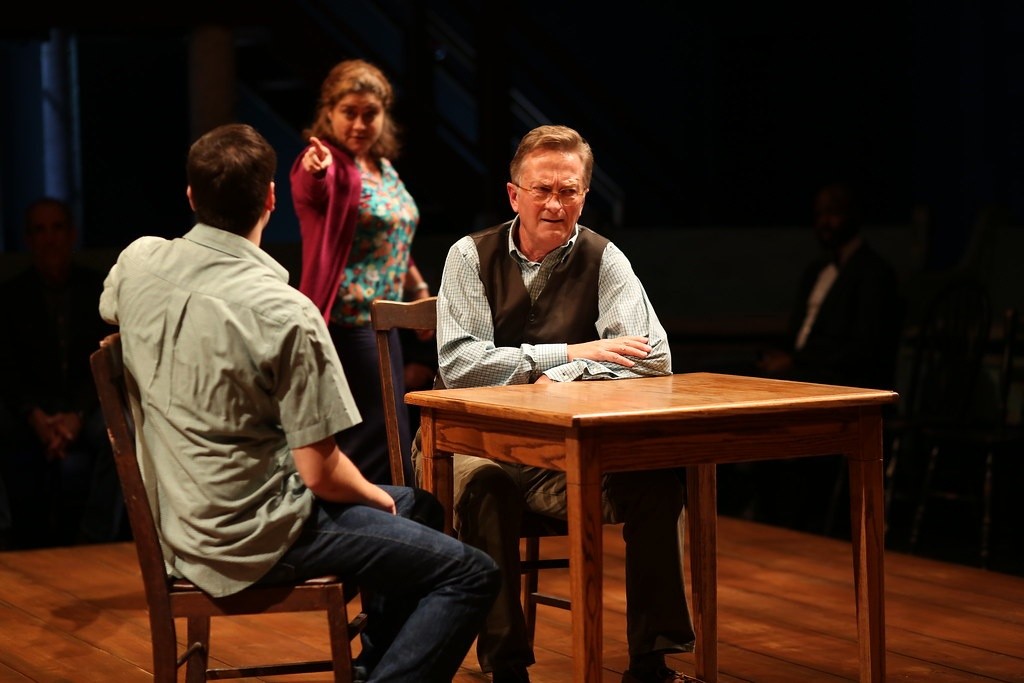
[404,372,901,683]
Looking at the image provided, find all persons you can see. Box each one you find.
[412,127,706,683]
[290,59,436,493]
[98,123,505,683]
[0,201,118,546]
[742,183,902,523]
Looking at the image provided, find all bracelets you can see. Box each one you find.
[413,282,428,293]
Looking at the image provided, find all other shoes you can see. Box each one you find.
[622,667,705,683]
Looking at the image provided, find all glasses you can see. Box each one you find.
[515,184,587,207]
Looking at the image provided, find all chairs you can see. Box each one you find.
[371,296,572,663]
[90,332,352,683]
